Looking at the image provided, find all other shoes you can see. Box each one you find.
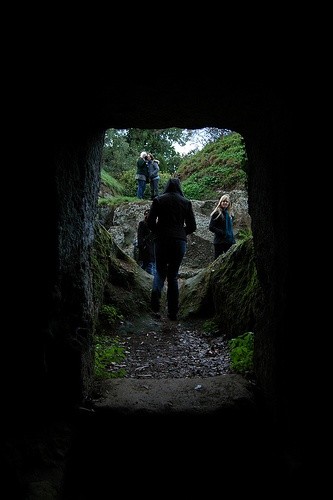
[150,310,161,320]
[167,313,177,321]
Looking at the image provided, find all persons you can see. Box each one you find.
[134,151,160,202]
[149,177,197,322]
[137,208,155,274]
[209,194,236,258]
